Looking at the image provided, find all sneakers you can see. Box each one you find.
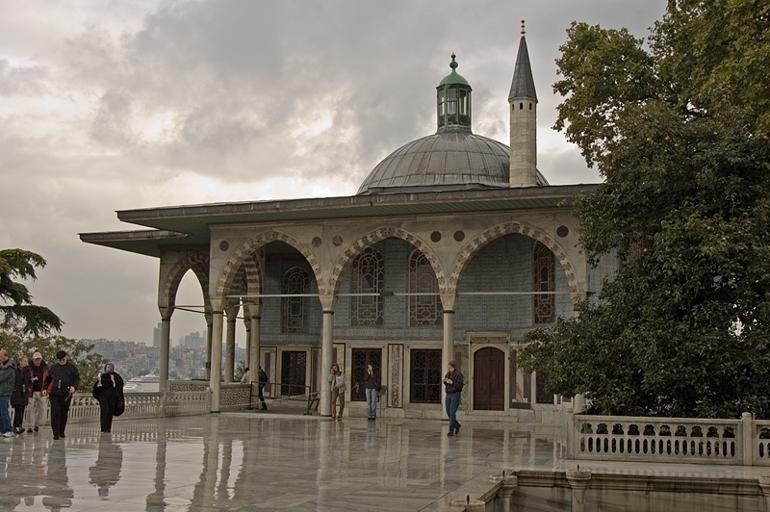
[260,407,267,410]
[446,423,462,437]
[332,412,343,418]
[0,425,39,438]
[53,432,65,440]
[367,417,376,420]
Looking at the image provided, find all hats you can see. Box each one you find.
[32,352,42,359]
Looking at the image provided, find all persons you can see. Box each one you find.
[0,431,75,512]
[0,348,16,439]
[41,350,80,441]
[87,432,123,500]
[23,351,50,433]
[327,362,346,419]
[443,360,465,436]
[240,367,252,383]
[363,363,380,420]
[92,361,119,433]
[247,364,269,410]
[9,357,30,436]
